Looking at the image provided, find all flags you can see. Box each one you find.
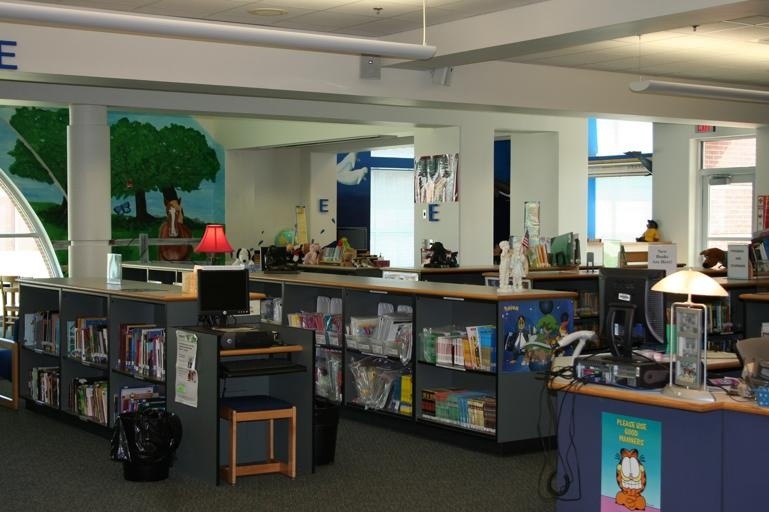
[521,230,529,250]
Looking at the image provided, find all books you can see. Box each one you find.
[23,309,166,426]
[418,323,498,437]
[387,369,412,415]
[371,311,412,342]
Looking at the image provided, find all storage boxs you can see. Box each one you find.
[618,242,674,267]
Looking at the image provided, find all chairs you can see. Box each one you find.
[0,276,34,338]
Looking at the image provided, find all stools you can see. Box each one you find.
[218,396,297,485]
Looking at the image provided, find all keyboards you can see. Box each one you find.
[223,358,296,372]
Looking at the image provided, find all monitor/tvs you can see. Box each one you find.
[337,227,367,251]
[598,266,666,363]
[259,246,287,271]
[198,269,250,328]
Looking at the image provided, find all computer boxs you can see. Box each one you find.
[573,356,669,388]
[192,327,272,349]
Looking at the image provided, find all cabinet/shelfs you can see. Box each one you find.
[482,267,768,350]
[20,268,250,432]
[251,269,578,457]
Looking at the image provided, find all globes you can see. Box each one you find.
[274,229,295,247]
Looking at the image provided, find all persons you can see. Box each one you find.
[498,241,511,290]
[505,315,534,367]
[510,235,529,291]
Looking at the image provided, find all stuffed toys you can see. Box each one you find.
[700,247,728,269]
[230,246,254,266]
[634,218,660,242]
[303,243,322,265]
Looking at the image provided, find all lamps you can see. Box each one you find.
[650,269,731,401]
[193,224,235,265]
[628,35,769,103]
[0,0,437,62]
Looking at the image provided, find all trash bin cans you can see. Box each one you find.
[117,409,176,482]
[312,395,338,466]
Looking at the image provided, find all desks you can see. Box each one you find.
[162,324,318,486]
[548,350,768,511]
